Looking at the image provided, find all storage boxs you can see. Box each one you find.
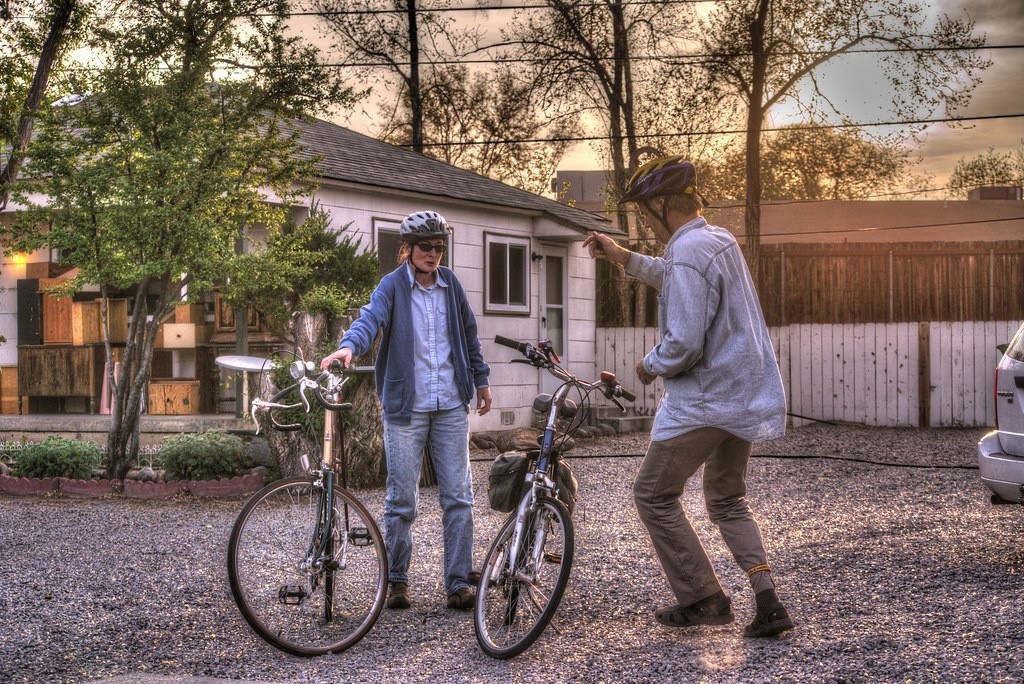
[17,263,208,349]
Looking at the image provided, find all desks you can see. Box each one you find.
[215,356,281,420]
[15,342,126,415]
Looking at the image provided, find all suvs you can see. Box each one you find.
[977,320,1024,506]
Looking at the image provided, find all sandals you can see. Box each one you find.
[654,597,735,627]
[744,603,793,636]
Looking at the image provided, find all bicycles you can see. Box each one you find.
[468,334,635,659]
[228,348,389,657]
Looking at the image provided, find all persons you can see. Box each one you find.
[321,210,492,611]
[582,155,793,638]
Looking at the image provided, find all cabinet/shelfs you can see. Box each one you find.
[147,381,200,414]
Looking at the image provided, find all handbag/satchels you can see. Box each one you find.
[552,459,578,523]
[488,450,530,513]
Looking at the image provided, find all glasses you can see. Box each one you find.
[415,242,448,253]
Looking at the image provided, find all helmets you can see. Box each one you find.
[616,155,697,204]
[399,211,451,244]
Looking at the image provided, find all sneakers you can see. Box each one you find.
[387,582,411,609]
[447,587,477,609]
